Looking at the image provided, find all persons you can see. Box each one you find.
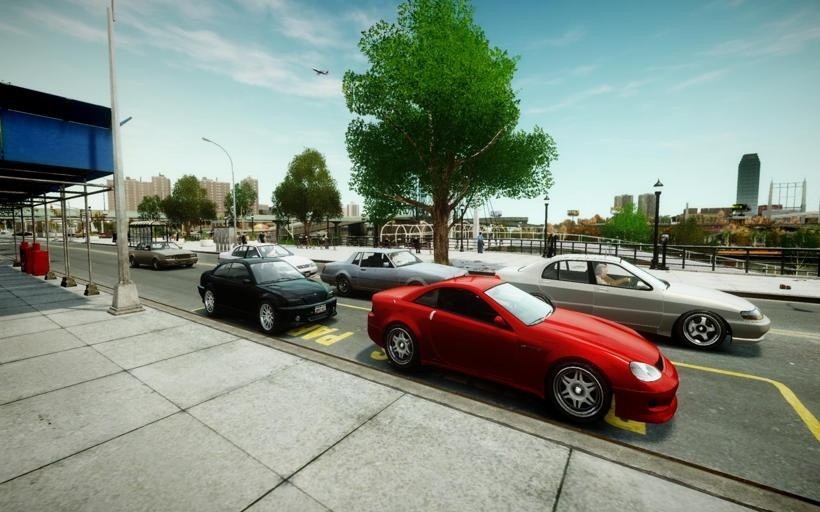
[242,232,248,244]
[596,264,610,286]
[382,235,391,248]
[601,263,632,287]
[258,232,266,243]
[414,238,421,253]
[478,232,484,253]
[543,233,559,258]
[299,232,330,249]
